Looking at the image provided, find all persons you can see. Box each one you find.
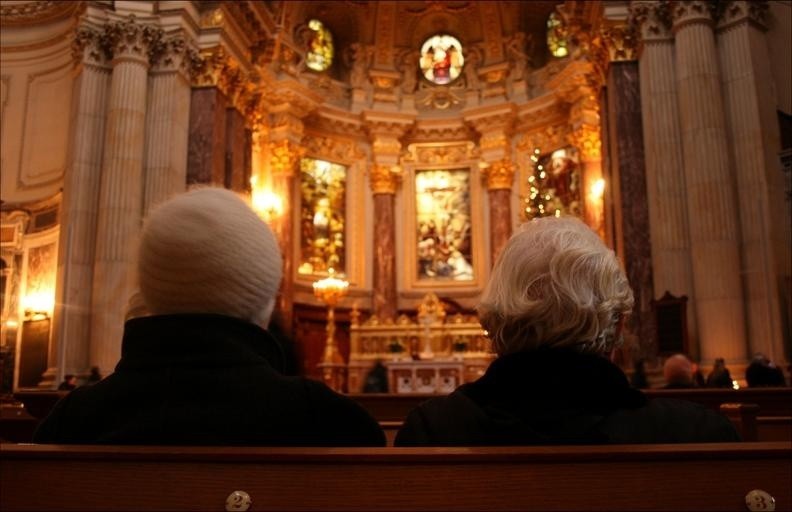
[57,374,76,390]
[2,351,13,390]
[629,358,649,388]
[361,361,389,394]
[87,366,103,382]
[662,346,790,389]
[32,183,387,446]
[391,212,739,444]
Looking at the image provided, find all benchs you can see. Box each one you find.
[1,353,791,512]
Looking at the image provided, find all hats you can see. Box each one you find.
[139,186,285,324]
